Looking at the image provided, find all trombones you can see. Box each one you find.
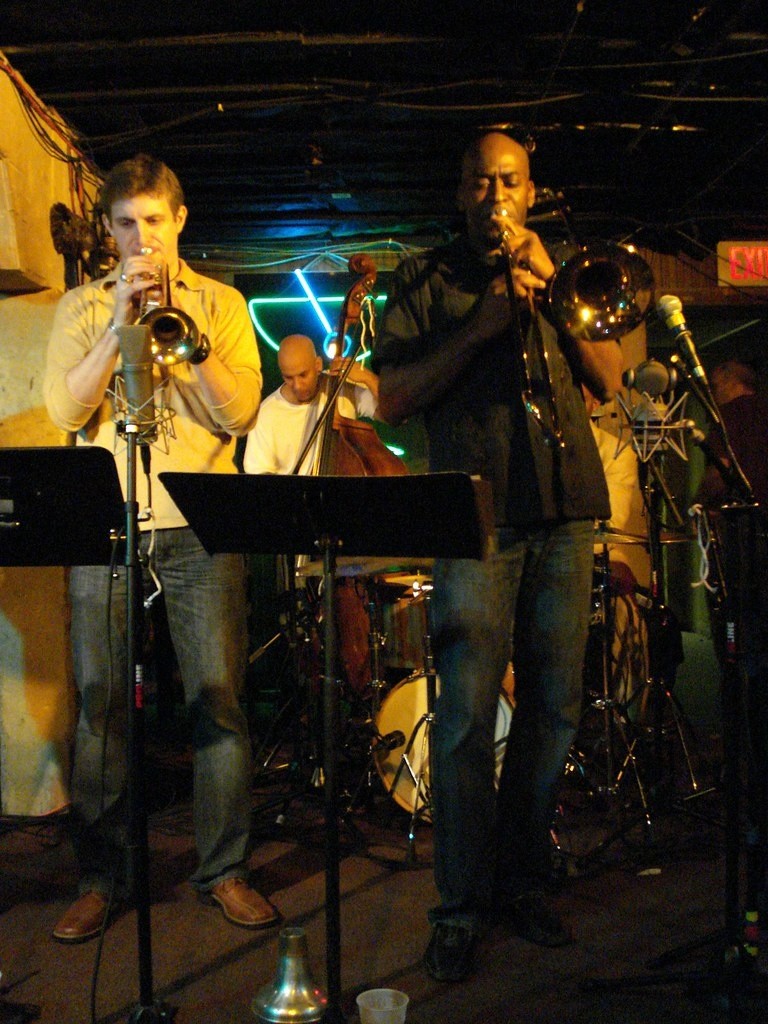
[492,204,657,449]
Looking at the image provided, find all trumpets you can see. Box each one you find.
[136,246,201,367]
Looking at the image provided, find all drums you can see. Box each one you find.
[374,672,515,825]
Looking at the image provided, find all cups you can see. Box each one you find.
[356,989,411,1024]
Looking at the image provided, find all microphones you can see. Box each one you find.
[657,293,708,386]
[371,729,405,753]
[116,324,157,435]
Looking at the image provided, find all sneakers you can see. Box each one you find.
[424,921,473,982]
[496,895,570,948]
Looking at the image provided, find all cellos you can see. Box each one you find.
[298,252,416,592]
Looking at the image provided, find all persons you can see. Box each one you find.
[683,358,767,726]
[574,374,640,754]
[41,153,284,950]
[370,129,636,981]
[243,333,410,718]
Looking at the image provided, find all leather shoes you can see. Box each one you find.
[209,876,279,929]
[53,889,133,941]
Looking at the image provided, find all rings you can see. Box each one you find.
[119,273,130,283]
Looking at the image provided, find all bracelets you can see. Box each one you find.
[186,333,213,365]
[107,318,122,336]
